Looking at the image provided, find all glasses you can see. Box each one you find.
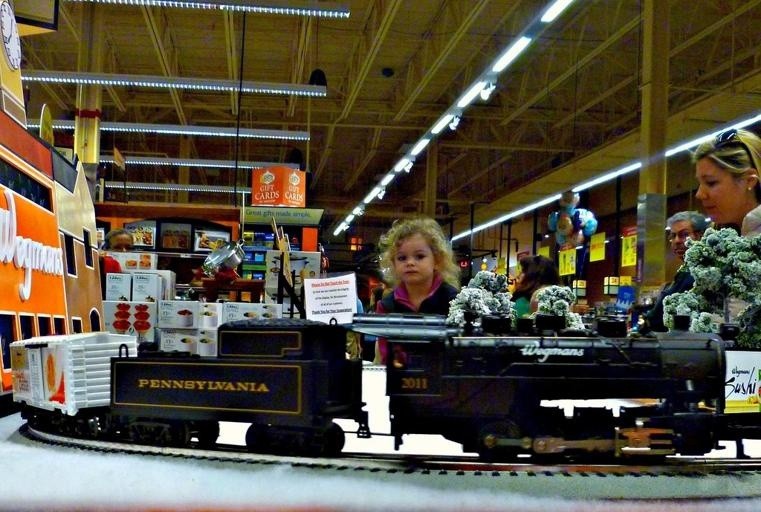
[714,129,758,170]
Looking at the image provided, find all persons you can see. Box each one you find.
[689,126,761,239]
[641,209,711,332]
[507,253,564,303]
[373,281,394,363]
[100,229,133,252]
[372,212,466,366]
[362,287,384,361]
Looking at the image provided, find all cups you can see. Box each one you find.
[177,309,219,358]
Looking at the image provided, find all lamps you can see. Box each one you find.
[82,3,354,18]
[22,117,311,143]
[103,180,252,194]
[332,0,574,237]
[95,154,302,173]
[19,68,326,99]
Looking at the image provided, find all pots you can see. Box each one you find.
[200,240,246,278]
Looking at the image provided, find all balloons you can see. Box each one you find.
[546,190,600,248]
